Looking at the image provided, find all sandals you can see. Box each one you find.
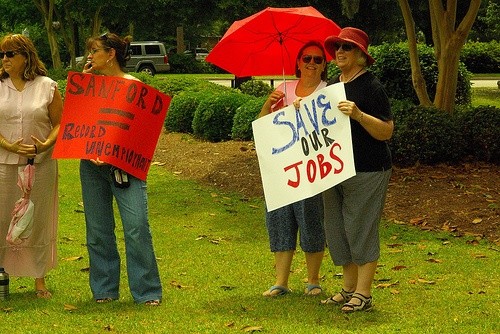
[340,292,373,312]
[320,288,356,305]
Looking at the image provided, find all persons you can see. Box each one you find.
[323,27,394,312]
[0,33,63,299]
[257,41,326,296]
[80,31,162,306]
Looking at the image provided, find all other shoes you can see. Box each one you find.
[96,298,113,303]
[145,300,159,306]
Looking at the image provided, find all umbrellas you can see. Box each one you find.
[205,6,343,105]
[6,145,37,245]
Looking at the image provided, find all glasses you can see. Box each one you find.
[334,44,357,51]
[0,50,23,58]
[301,55,324,64]
[88,47,111,54]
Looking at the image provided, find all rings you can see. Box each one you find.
[345,107,348,110]
[293,101,295,103]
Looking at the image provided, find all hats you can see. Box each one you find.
[325,26,375,64]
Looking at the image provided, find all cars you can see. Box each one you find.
[184,47,210,62]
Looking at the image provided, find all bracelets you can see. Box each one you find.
[359,113,364,122]
[1,137,6,147]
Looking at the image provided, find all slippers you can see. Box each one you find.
[34,288,52,300]
[307,284,323,295]
[263,286,289,297]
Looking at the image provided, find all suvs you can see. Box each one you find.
[70,40,170,77]
